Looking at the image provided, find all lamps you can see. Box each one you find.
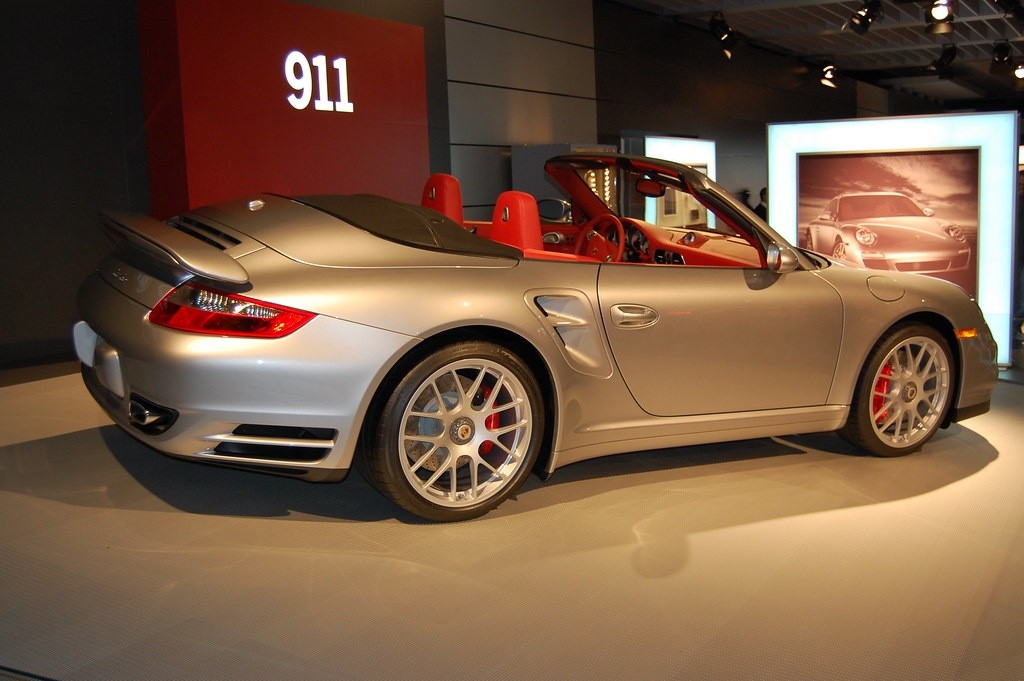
[850,0,880,34]
[926,43,956,80]
[708,10,745,64]
[819,62,840,88]
[925,0,954,34]
[990,38,1015,67]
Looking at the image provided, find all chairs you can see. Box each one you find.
[421,173,463,229]
[491,191,598,262]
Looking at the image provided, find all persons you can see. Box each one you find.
[753,187,767,221]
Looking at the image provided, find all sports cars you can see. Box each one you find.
[805,190,973,276]
[72,152,1000,522]
